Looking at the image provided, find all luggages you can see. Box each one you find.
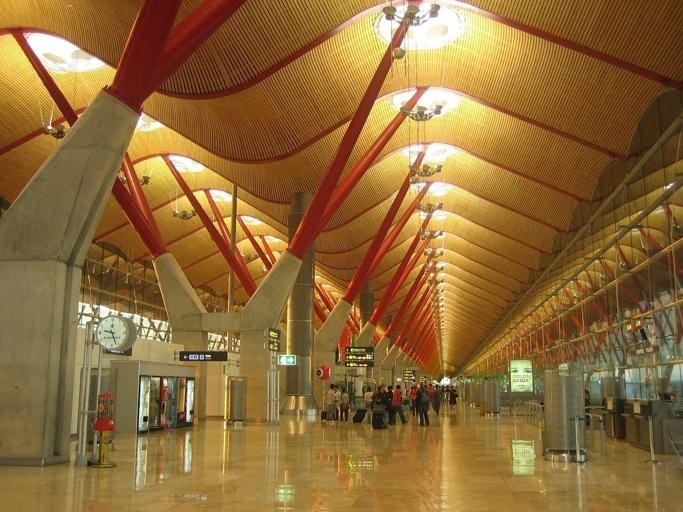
[372,410,385,429]
[320,403,328,421]
[353,409,368,424]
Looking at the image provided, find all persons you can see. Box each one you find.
[323,381,458,426]
[584,390,591,425]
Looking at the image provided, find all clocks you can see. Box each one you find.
[95,315,130,351]
